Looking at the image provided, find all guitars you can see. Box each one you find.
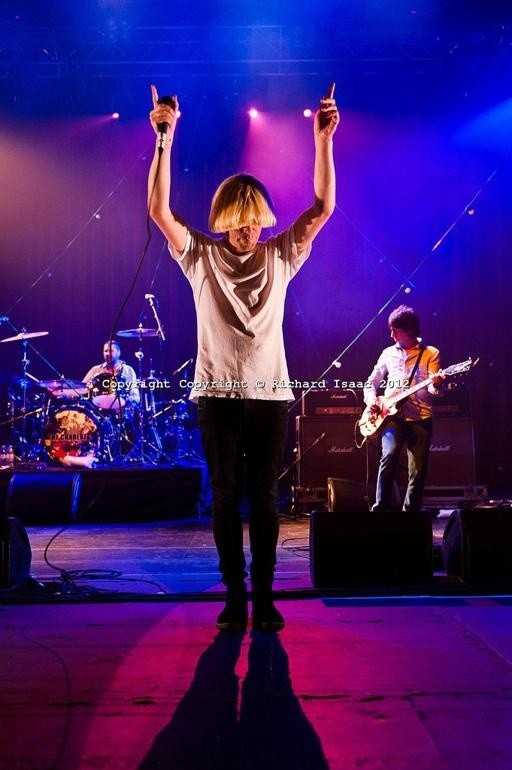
[359,357,481,437]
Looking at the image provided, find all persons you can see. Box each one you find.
[360,304,449,512]
[80,339,142,419]
[142,74,340,634]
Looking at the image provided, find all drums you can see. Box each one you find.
[43,403,103,467]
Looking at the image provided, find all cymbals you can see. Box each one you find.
[116,328,161,339]
[1,332,50,342]
[37,380,88,388]
[92,393,124,409]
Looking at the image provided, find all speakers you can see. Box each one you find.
[309,510,433,590]
[442,509,512,590]
[0,515,31,593]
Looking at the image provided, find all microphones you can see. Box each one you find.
[158,96,173,155]
[144,294,155,300]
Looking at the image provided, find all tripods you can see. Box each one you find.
[279,437,323,522]
[0,338,207,468]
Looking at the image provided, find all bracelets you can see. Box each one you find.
[155,135,174,150]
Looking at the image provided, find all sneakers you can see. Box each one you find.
[252,602,284,628]
[216,602,247,629]
